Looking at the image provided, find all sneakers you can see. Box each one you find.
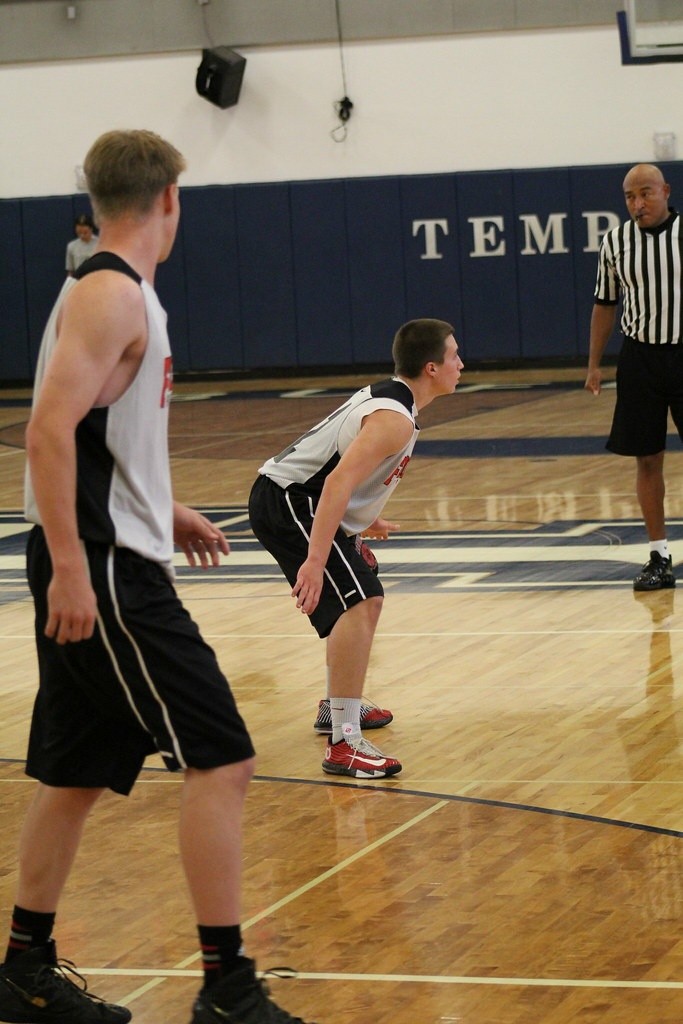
[633,550,676,590]
[315,727,394,754]
[326,777,402,809]
[321,734,402,778]
[188,966,315,1024]
[0,958,133,1024]
[314,695,393,733]
[633,588,675,622]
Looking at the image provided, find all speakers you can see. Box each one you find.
[195,44,246,109]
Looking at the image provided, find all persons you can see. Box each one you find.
[584,164,683,592]
[0,131,319,1024]
[247,321,463,779]
